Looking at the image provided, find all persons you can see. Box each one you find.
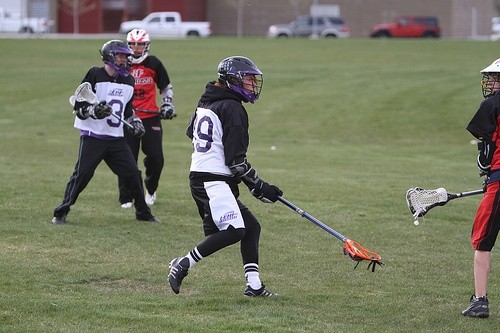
[48,39,163,224]
[461,56,500,318]
[164,55,283,300]
[108,27,177,209]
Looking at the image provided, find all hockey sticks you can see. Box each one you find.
[278,197,383,263]
[75,81,136,131]
[406,187,484,218]
[133,108,178,117]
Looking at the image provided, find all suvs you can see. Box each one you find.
[267,14,350,39]
[369,16,442,39]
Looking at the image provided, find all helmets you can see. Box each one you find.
[125,28,151,65]
[480,58,500,100]
[218,56,263,105]
[99,39,134,80]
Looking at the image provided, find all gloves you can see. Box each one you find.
[476,154,491,175]
[252,179,284,204]
[88,100,113,120]
[159,102,175,120]
[128,117,145,138]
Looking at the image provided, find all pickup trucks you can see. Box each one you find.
[119,11,213,38]
[0,8,50,35]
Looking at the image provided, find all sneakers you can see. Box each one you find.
[244,281,278,298]
[167,257,189,296]
[145,189,157,205]
[461,294,490,318]
[121,199,134,208]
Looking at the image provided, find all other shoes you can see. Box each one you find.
[149,216,161,223]
[51,215,67,225]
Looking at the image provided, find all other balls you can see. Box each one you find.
[271,146,276,150]
[413,221,420,226]
